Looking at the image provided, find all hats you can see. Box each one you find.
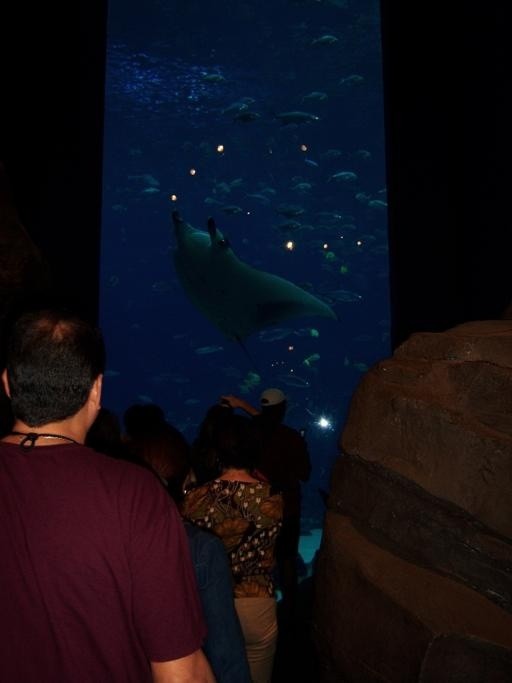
[258,387,287,407]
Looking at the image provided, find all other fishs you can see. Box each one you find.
[100,90,390,434]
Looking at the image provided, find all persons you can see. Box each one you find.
[1,313,215,682]
[84,385,306,683]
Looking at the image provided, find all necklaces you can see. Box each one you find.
[9,431,80,448]
